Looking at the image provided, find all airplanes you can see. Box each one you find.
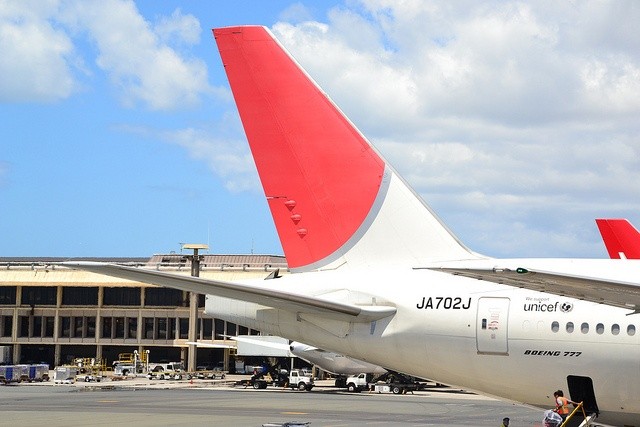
[58,26,640,425]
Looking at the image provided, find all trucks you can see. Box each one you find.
[54,366,76,383]
[0,364,49,382]
[148,362,181,374]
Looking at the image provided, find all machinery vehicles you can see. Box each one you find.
[234,362,314,390]
[346,372,426,394]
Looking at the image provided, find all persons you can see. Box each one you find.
[553,389,580,422]
[503,417,510,426]
